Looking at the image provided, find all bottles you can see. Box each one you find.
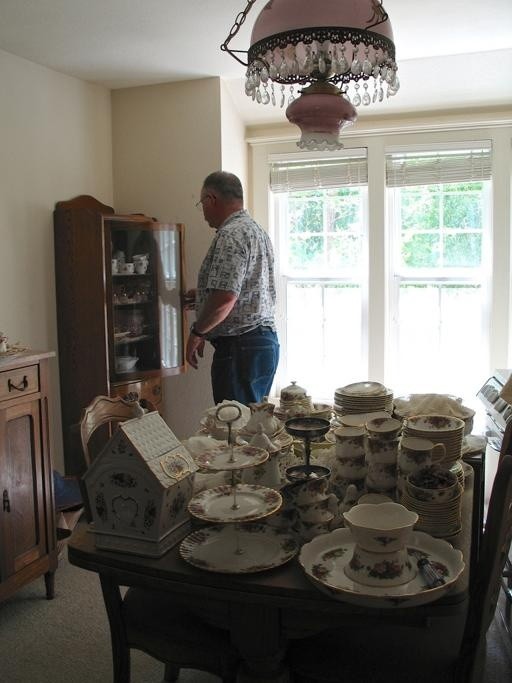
[111,259,117,273]
[280,381,306,401]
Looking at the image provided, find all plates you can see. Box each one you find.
[272,403,332,421]
[325,381,394,444]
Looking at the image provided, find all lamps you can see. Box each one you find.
[221,0,400,151]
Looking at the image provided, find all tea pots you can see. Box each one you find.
[240,423,292,491]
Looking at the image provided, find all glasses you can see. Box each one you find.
[195,194,216,211]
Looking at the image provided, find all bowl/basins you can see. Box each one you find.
[115,356,139,370]
[396,415,465,542]
[394,406,476,436]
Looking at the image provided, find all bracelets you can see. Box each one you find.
[189,321,206,339]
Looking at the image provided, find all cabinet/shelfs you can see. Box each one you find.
[54,195,190,476]
[0,357,59,605]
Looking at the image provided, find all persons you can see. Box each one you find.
[182,171,281,413]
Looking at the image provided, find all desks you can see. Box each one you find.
[68,459,475,683]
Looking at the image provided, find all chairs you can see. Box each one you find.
[284,455,512,683]
[68,395,242,683]
[500,422,512,462]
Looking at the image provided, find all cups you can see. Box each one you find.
[118,253,149,274]
[334,427,401,502]
